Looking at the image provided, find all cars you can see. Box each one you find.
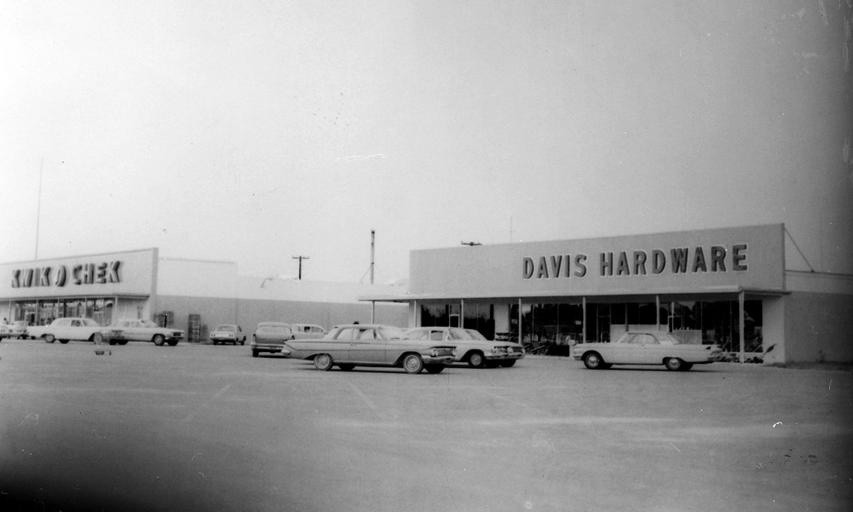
[572,330,724,372]
[209,324,247,345]
[250,322,525,374]
[0,317,185,346]
